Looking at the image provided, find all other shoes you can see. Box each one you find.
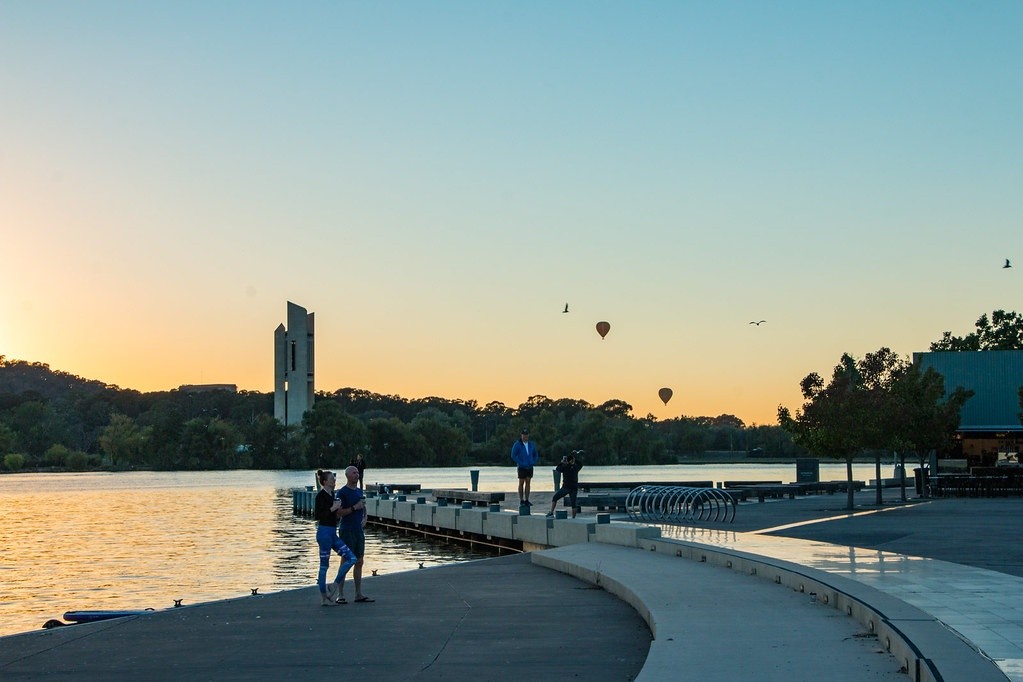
[328,584,336,598]
[323,597,334,606]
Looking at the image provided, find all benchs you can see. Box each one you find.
[564,481,865,513]
[433,489,505,507]
[367,485,420,495]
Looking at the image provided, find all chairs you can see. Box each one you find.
[937,472,1022,498]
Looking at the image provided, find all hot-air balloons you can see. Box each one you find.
[596,322,610,339]
[659,388,673,406]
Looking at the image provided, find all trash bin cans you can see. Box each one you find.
[913,467,930,497]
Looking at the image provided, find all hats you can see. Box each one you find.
[521,429,529,434]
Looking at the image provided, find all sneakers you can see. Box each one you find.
[545,513,554,517]
[525,501,532,506]
[520,500,526,506]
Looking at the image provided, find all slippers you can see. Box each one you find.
[336,598,348,604]
[354,596,375,602]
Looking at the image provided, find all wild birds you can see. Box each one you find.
[749,320,766,326]
[562,302,569,313]
[1003,259,1012,269]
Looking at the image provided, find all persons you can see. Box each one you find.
[350,454,366,491]
[333,467,373,604]
[544,453,579,521]
[510,428,540,506]
[313,469,356,606]
